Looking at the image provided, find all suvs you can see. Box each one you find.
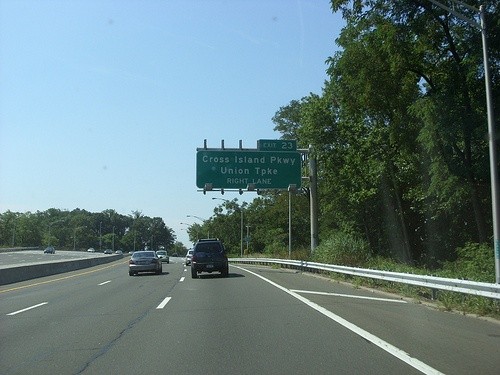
[191,238,228,278]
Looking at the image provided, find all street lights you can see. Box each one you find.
[246,226,251,258]
[186,215,210,239]
[212,197,243,258]
[73,226,87,250]
[180,222,198,240]
[49,219,65,247]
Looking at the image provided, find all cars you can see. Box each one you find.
[43,247,55,254]
[104,249,112,254]
[129,251,133,254]
[88,247,95,252]
[155,250,169,264]
[116,250,122,254]
[185,250,194,266]
[172,253,178,257]
[128,250,163,276]
